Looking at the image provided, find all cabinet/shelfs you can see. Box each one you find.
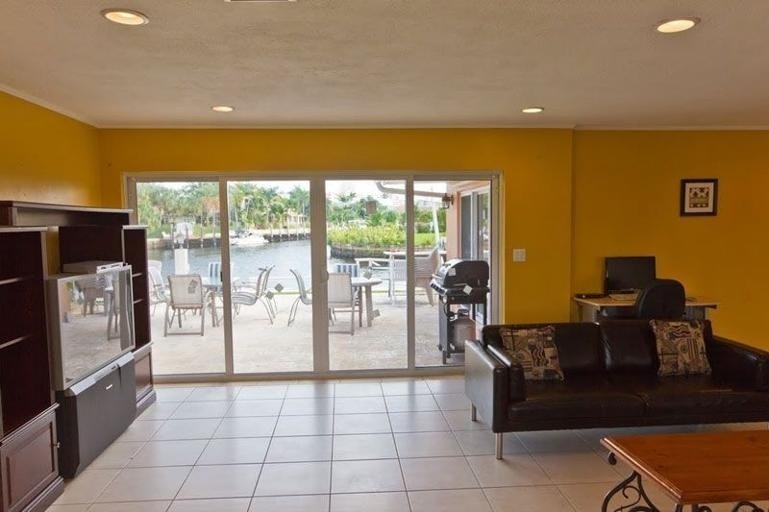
[57,223,157,419]
[1,221,66,512]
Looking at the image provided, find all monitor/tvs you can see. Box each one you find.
[44,264,138,393]
[604,256,657,294]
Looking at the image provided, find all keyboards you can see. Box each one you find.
[609,292,636,302]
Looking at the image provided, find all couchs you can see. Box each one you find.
[455,318,769,460]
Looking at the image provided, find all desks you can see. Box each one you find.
[348,275,383,328]
[572,291,718,322]
[429,279,490,364]
[55,351,138,480]
[382,250,448,298]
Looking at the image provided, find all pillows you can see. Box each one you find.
[647,318,715,377]
[498,324,569,385]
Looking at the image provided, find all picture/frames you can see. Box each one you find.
[679,177,718,217]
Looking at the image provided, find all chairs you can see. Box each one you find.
[146,260,276,336]
[287,264,363,337]
[630,278,685,320]
[392,246,440,306]
